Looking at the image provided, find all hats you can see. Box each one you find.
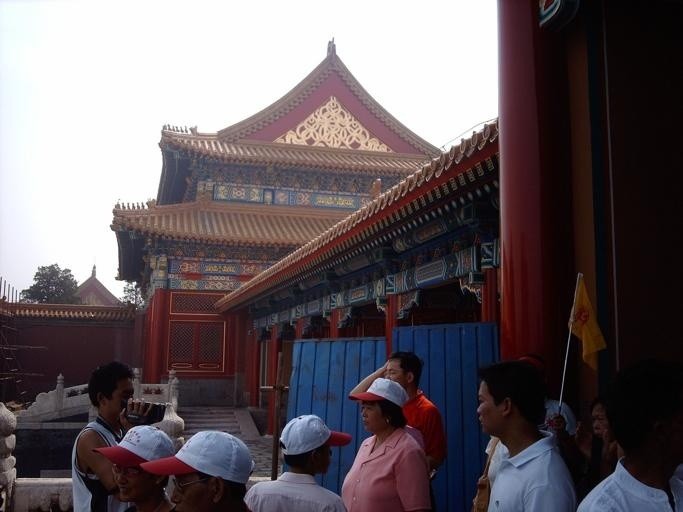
[349,377,409,407]
[140,430,251,483]
[279,415,352,456]
[91,425,175,470]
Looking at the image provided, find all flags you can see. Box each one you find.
[567,273,606,369]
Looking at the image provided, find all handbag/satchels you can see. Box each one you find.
[473,475,490,512]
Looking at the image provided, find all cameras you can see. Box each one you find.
[121,399,165,427]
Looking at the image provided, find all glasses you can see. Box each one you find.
[172,477,212,493]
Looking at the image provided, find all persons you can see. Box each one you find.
[140,431,255,512]
[576,359,683,512]
[342,378,431,512]
[71,361,154,512]
[92,426,175,512]
[244,414,353,512]
[477,357,583,512]
[350,351,447,480]
